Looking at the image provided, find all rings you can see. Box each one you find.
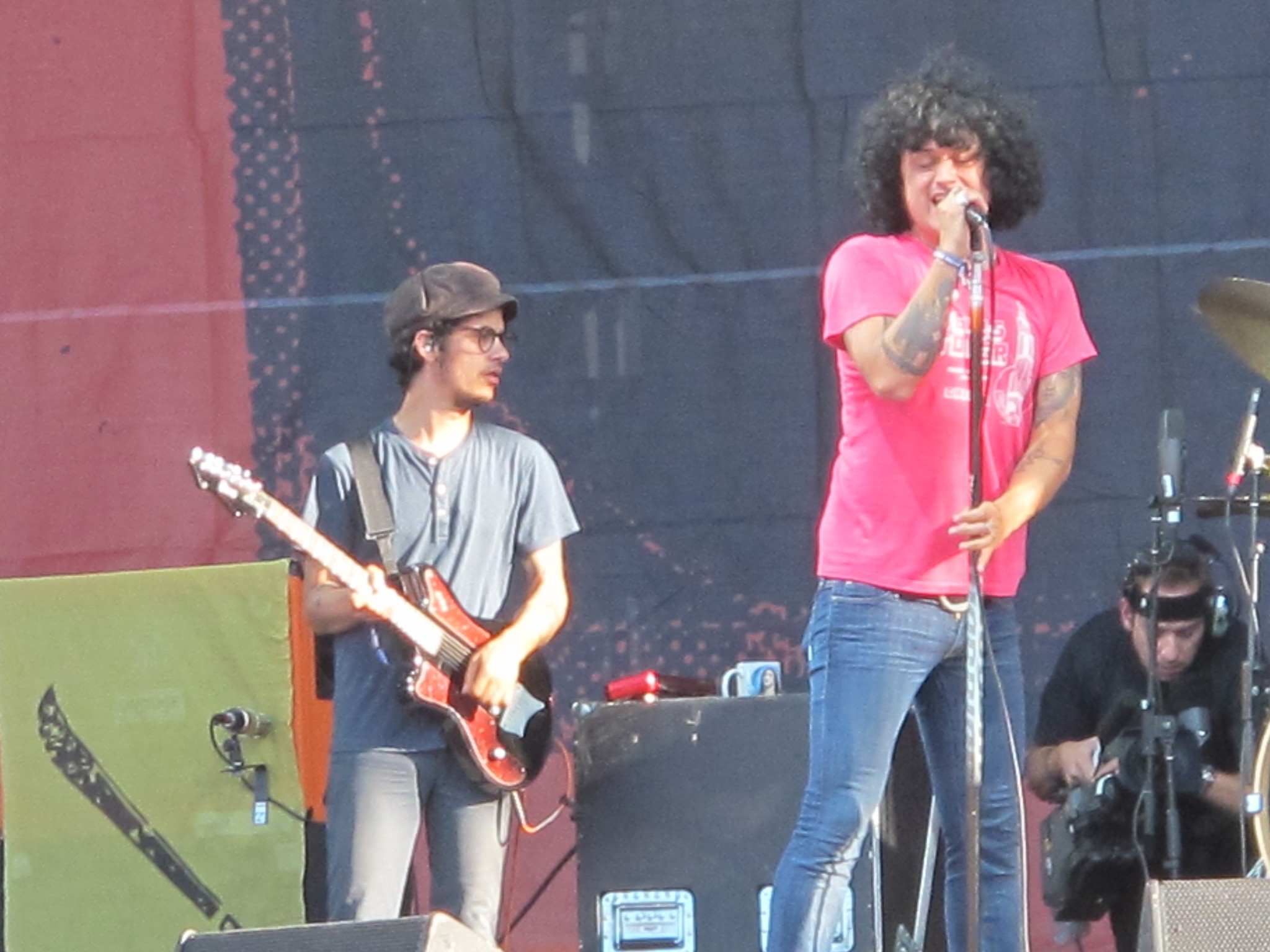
[953,189,970,206]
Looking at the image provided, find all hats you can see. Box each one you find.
[385,261,518,347]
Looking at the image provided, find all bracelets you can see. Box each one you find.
[1200,763,1215,784]
[934,248,964,268]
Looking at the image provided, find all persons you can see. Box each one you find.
[1020,538,1270,952]
[293,262,580,942]
[769,53,1097,952]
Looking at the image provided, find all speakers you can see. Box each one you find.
[1134,873,1267,952]
[575,698,952,952]
[174,910,501,952]
[0,560,333,952]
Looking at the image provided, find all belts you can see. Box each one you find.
[900,590,970,612]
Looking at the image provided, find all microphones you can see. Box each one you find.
[939,193,985,230]
[1225,384,1263,497]
[214,708,271,736]
[1154,405,1183,504]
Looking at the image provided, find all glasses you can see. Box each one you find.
[428,325,519,353]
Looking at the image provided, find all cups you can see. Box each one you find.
[720,661,782,698]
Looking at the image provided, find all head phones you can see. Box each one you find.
[1120,538,1239,640]
